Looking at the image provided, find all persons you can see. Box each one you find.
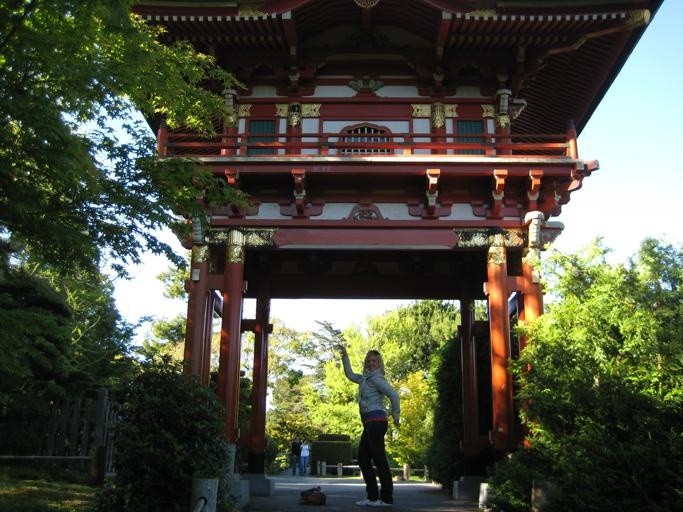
[291,436,302,476]
[300,439,310,476]
[334,342,400,508]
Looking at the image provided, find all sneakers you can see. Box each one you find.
[367,498,392,506]
[355,498,370,506]
